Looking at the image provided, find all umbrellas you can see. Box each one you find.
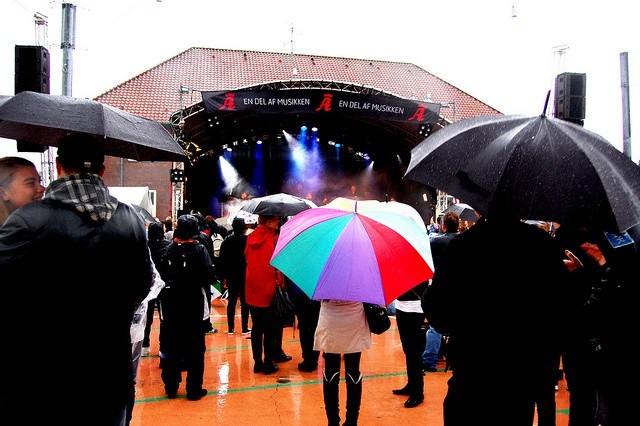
[403,87,635,238]
[270,197,435,309]
[1,88,191,163]
[239,192,317,219]
[441,203,477,223]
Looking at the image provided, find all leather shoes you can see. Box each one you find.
[406,396,423,407]
[275,346,292,363]
[393,388,411,395]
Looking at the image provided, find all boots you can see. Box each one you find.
[343,372,361,426]
[324,369,339,426]
[299,348,319,372]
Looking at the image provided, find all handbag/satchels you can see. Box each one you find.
[264,286,290,325]
[363,302,390,334]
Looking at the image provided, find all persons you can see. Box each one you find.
[275,288,291,363]
[422,208,553,425]
[288,280,318,373]
[422,210,460,373]
[147,216,209,400]
[139,224,169,358]
[2,131,153,425]
[243,214,280,372]
[218,217,253,337]
[196,212,213,333]
[312,298,374,425]
[562,247,638,425]
[390,281,425,408]
[1,156,44,231]
[128,243,166,381]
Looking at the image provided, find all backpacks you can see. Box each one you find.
[210,225,224,257]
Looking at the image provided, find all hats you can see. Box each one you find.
[174,215,200,240]
[57,134,104,172]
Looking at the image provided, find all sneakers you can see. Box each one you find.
[264,366,279,374]
[254,363,263,372]
[168,392,178,399]
[187,389,207,400]
[141,347,149,357]
[205,329,218,335]
[242,329,252,334]
[228,329,235,334]
